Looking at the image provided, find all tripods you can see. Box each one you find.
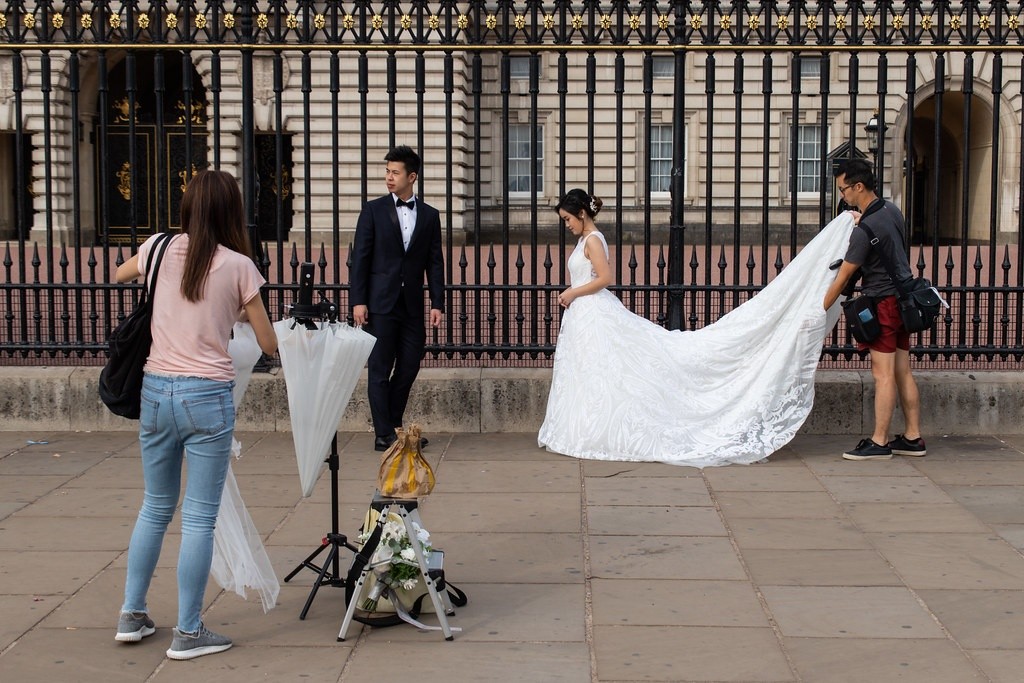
[284,431,358,623]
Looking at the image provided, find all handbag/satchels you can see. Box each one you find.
[356,561,447,613]
[98,231,177,421]
[896,278,943,333]
[839,293,881,346]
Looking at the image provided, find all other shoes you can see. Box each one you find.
[376,435,397,452]
[419,437,431,449]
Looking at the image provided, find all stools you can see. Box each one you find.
[337,488,454,642]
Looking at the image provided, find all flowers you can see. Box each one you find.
[359,520,433,613]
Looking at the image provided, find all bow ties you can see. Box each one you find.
[396,198,415,210]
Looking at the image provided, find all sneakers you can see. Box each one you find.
[842,438,893,460]
[115,611,156,642]
[167,621,232,660]
[887,433,927,456]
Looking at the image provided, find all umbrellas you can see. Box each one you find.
[273,301,377,497]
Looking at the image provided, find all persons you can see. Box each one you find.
[824,159,927,461]
[114,171,280,658]
[347,145,446,450]
[538,188,613,461]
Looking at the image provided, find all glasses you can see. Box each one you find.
[839,181,860,194]
